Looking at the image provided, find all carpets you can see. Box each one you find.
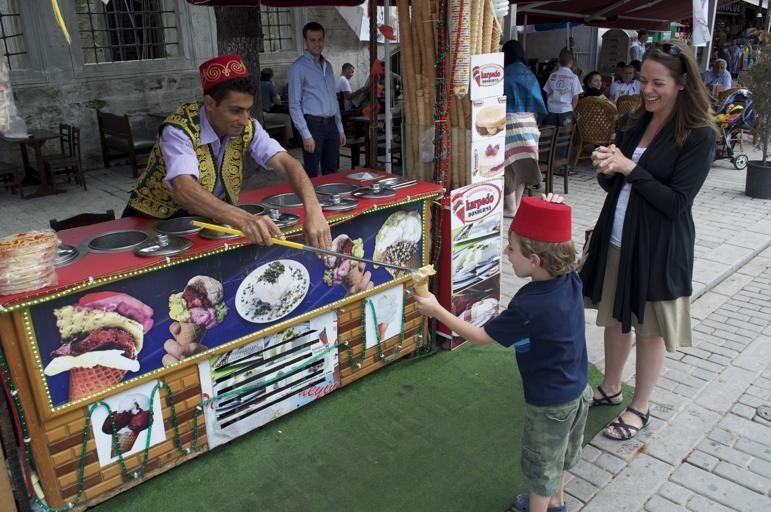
[80,339,636,512]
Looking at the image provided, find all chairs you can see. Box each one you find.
[336,91,360,138]
[44,125,87,194]
[49,210,116,232]
[550,123,577,194]
[0,163,25,200]
[528,127,557,196]
[720,88,747,151]
[617,95,638,115]
[574,96,618,169]
[43,123,79,184]
[262,113,289,147]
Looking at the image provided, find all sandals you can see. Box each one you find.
[589,385,625,408]
[604,406,652,441]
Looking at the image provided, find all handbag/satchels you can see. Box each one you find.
[578,231,602,310]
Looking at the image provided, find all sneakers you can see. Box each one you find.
[515,493,568,512]
[504,209,517,218]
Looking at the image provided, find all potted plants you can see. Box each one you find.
[733,12,771,200]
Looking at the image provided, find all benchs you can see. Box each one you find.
[96,109,156,177]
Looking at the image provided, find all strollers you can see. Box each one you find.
[713,88,757,169]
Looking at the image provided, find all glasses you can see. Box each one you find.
[645,40,681,57]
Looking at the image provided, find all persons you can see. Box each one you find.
[344,262,375,296]
[613,60,625,83]
[122,53,333,261]
[630,29,648,62]
[543,51,583,136]
[580,39,721,440]
[501,40,548,218]
[162,320,208,366]
[288,21,347,178]
[411,193,593,511]
[560,38,575,59]
[260,67,285,113]
[336,62,368,115]
[727,39,742,74]
[583,70,618,109]
[610,64,641,102]
[701,58,732,93]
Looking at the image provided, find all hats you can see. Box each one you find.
[511,194,573,244]
[198,53,247,88]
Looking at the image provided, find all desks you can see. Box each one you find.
[350,110,400,168]
[1,128,68,199]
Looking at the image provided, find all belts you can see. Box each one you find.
[305,114,334,123]
